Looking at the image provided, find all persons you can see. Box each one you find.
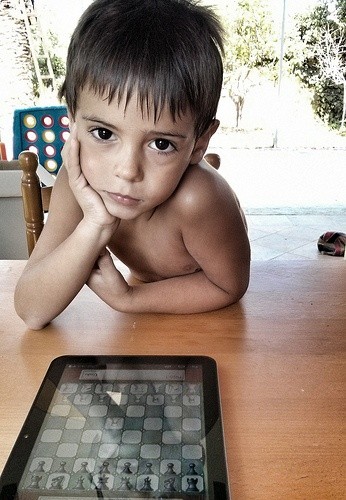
[13,0,251,330]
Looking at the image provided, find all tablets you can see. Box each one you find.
[0,354,231,500]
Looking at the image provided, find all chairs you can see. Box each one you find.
[0,153,46,259]
[13,107,70,177]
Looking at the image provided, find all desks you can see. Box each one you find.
[0,249,346,500]
